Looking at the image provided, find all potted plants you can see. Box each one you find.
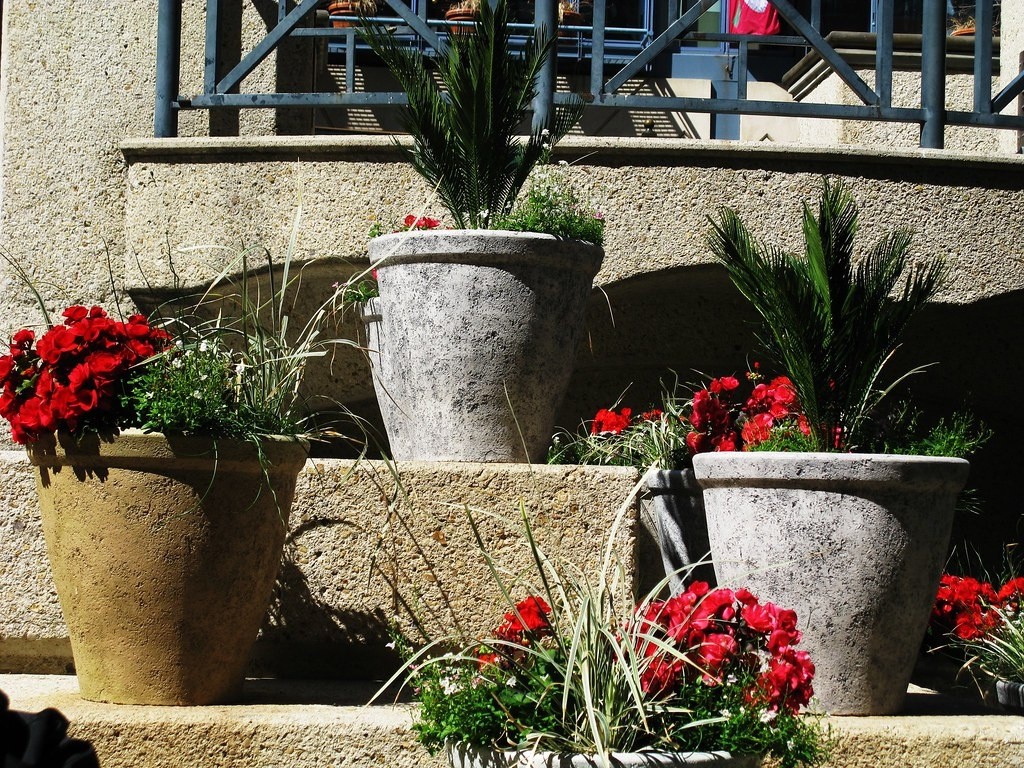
[359,0,605,463]
[691,170,997,716]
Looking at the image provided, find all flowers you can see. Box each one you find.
[379,577,838,768]
[545,360,856,471]
[332,129,609,305]
[925,540,1024,701]
[0,172,449,528]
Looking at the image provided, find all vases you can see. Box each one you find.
[329,2,582,38]
[645,467,717,600]
[26,428,313,707]
[452,740,735,768]
[995,679,1024,715]
[367,230,604,462]
[356,296,395,461]
